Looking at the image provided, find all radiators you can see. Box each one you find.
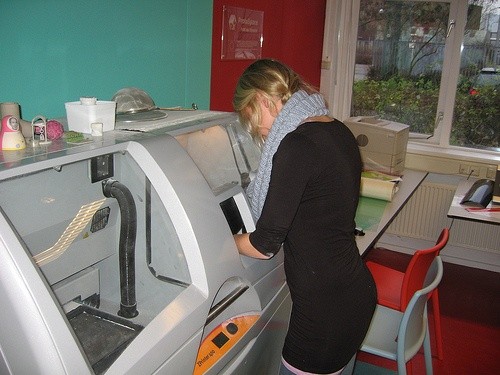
[385,185,500,254]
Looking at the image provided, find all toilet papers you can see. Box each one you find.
[359,176,398,202]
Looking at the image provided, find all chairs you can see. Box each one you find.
[357,228,449,375]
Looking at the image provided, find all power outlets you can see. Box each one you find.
[469,166,479,175]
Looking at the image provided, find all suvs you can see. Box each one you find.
[469,67,500,95]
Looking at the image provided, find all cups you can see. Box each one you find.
[79,96,97,105]
[91,123,102,137]
[0,102,20,122]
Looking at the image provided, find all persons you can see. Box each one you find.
[234,58,378,375]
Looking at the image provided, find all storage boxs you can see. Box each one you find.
[65,100,117,132]
[343,115,408,173]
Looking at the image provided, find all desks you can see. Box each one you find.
[355,170,430,258]
[447,180,500,226]
